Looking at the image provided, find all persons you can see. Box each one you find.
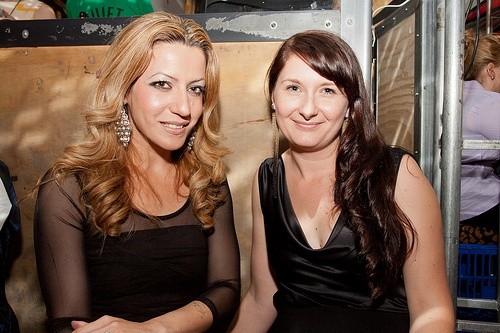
[219,30,456,332]
[28,9,243,333]
[0,138,22,333]
[437,27,500,263]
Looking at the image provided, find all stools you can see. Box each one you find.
[459,225,499,321]
